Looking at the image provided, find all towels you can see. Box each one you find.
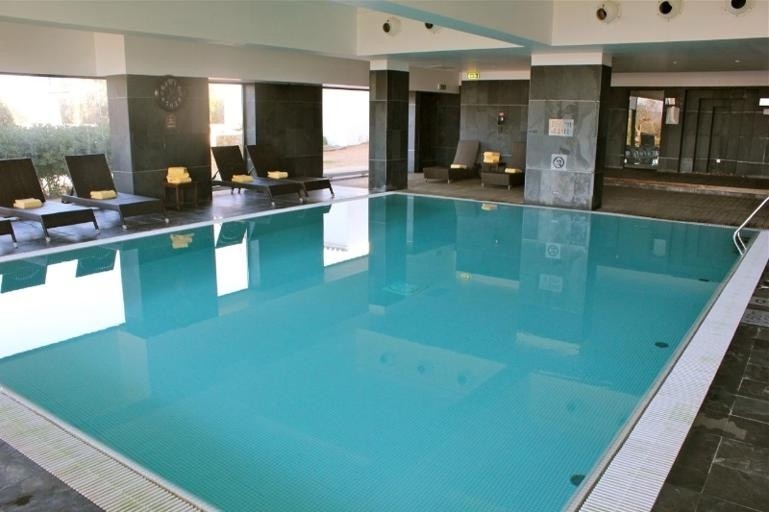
[11,197,43,212]
[502,168,522,174]
[229,173,255,184]
[163,166,192,185]
[265,170,289,181]
[482,150,501,163]
[87,189,118,200]
[449,163,466,169]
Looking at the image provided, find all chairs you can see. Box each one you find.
[481,138,527,191]
[0,155,106,244]
[57,149,174,231]
[244,143,336,202]
[420,139,482,185]
[207,144,306,207]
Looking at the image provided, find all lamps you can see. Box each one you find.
[657,0,683,18]
[497,111,506,126]
[424,20,440,32]
[595,0,624,25]
[379,17,403,38]
[729,0,755,17]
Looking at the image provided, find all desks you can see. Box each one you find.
[477,162,507,177]
[160,178,203,211]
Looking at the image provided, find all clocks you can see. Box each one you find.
[154,77,187,112]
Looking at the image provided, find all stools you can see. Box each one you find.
[0,217,21,249]
